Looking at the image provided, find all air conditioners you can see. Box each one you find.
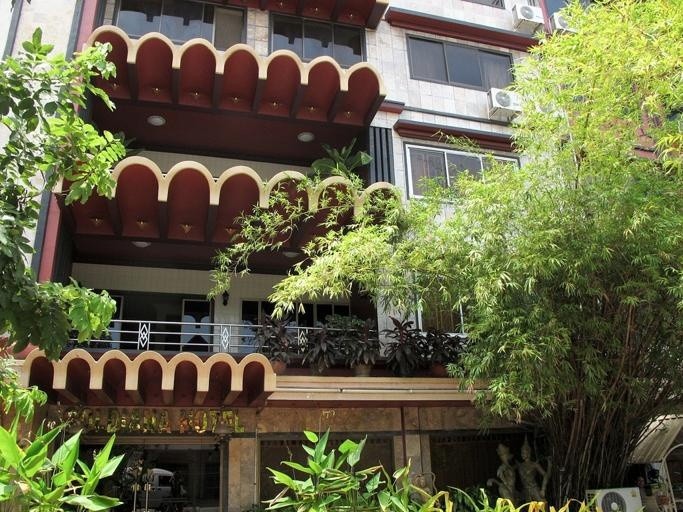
[583,486,644,512]
[486,86,525,121]
[512,4,577,34]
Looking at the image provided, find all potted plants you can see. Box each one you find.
[251,310,461,378]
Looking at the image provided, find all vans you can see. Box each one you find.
[122,466,187,511]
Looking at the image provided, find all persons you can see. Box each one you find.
[167,470,187,512]
